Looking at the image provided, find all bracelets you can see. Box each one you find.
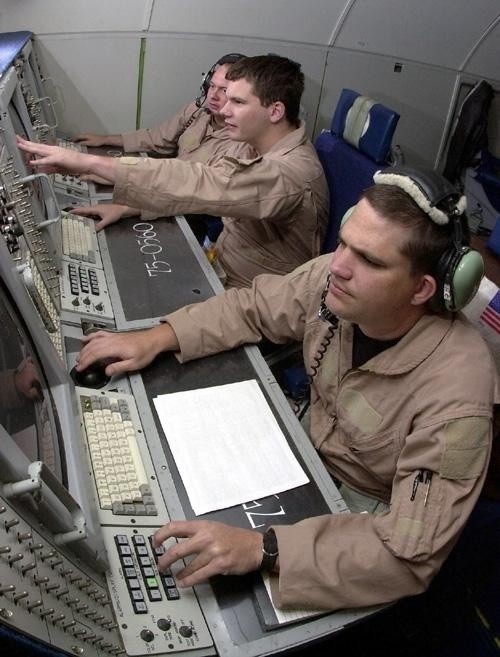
[260,533,278,570]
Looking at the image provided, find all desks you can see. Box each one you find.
[45,113,410,657]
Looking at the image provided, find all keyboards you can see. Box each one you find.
[75,385,173,527]
[57,138,82,152]
[61,210,105,270]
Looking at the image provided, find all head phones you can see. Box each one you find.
[200,53,247,95]
[339,164,486,316]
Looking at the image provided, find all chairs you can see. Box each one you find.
[306,86,402,255]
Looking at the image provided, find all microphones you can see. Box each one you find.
[195,95,211,115]
[322,309,339,326]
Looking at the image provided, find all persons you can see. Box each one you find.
[76,165,495,608]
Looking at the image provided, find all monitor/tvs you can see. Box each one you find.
[1,231,110,573]
[0,66,63,252]
[21,39,56,146]
